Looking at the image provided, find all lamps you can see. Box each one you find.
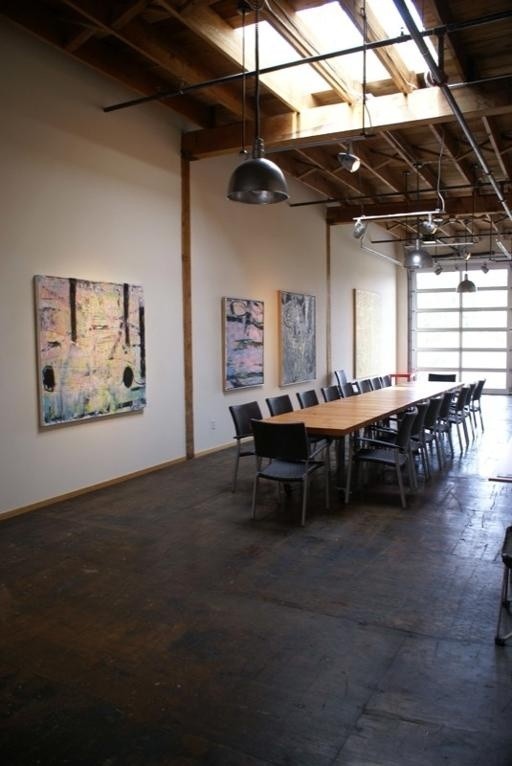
[435,262,443,275]
[481,263,489,274]
[353,221,366,238]
[403,236,434,268]
[423,214,437,234]
[337,142,361,173]
[226,0,291,204]
[457,260,476,292]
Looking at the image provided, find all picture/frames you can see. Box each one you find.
[32,274,145,428]
[222,297,264,391]
[354,288,385,379]
[278,290,317,387]
